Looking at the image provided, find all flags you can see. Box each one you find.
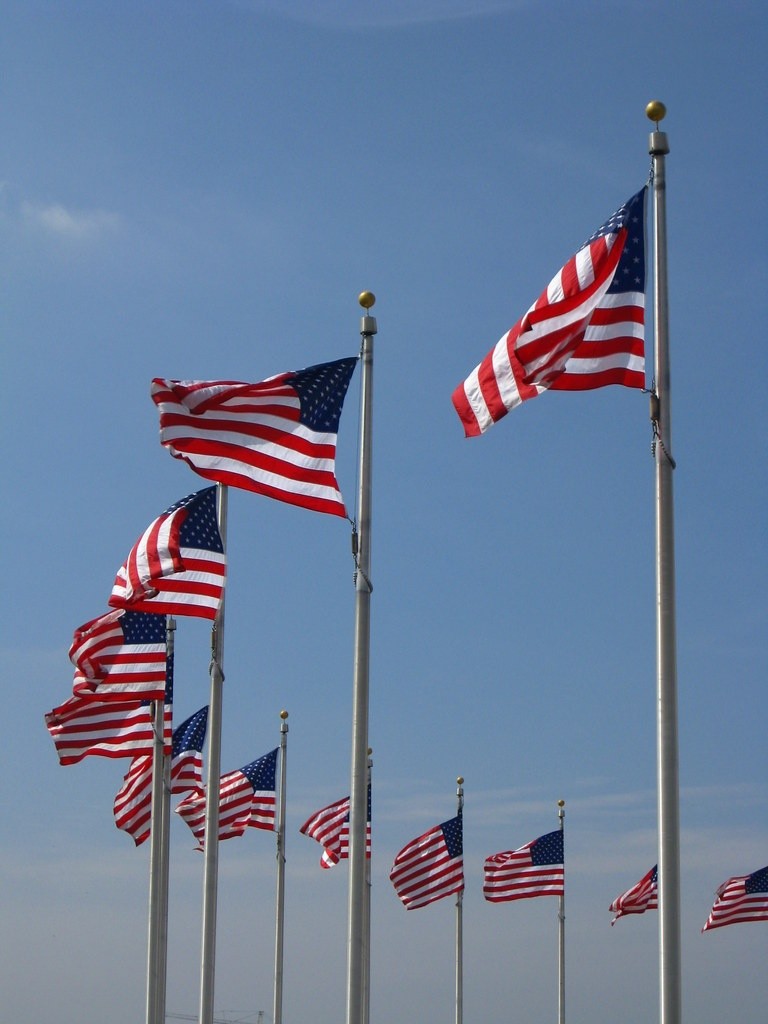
[44,486,768,933]
[152,356,356,525]
[450,179,648,439]
